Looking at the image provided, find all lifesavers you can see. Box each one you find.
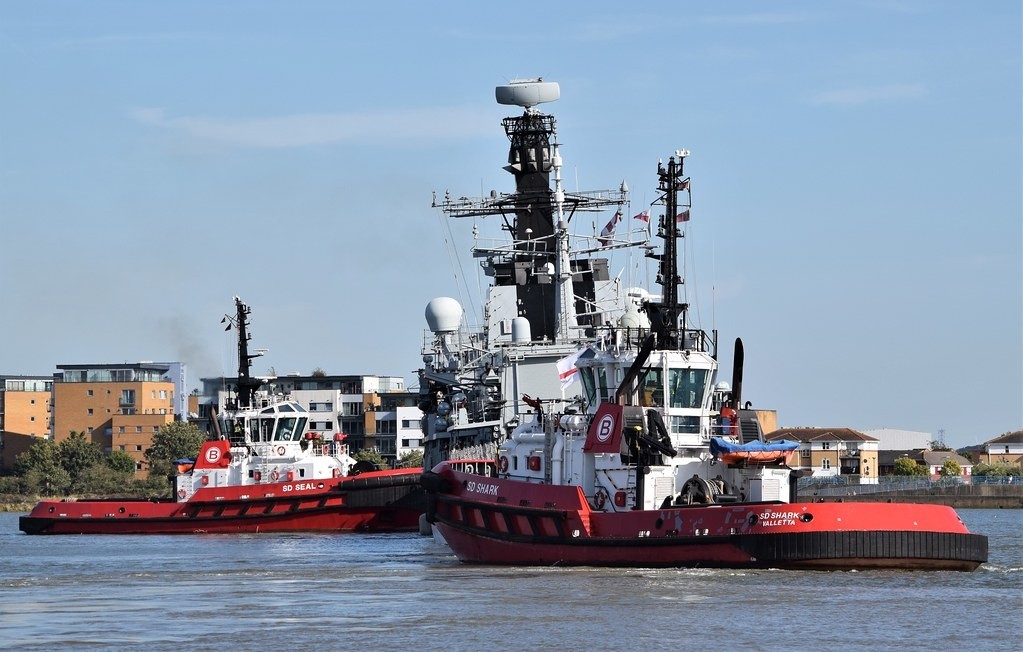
[278,446,286,455]
[499,456,508,472]
[594,492,605,508]
[271,470,280,480]
[178,488,187,499]
[340,445,344,453]
[323,445,328,454]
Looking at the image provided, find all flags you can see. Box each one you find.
[556,347,597,388]
[677,210,689,222]
[633,208,649,222]
[598,212,617,246]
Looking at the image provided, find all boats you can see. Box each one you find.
[413,75,987,572]
[19,295,420,533]
[711,436,798,465]
